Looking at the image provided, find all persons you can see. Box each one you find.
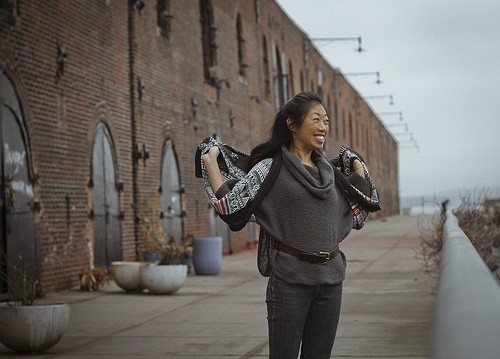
[200,91,372,359]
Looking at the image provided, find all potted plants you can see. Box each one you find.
[78,195,193,295]
[0,256,70,355]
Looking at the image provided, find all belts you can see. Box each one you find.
[274,241,339,265]
